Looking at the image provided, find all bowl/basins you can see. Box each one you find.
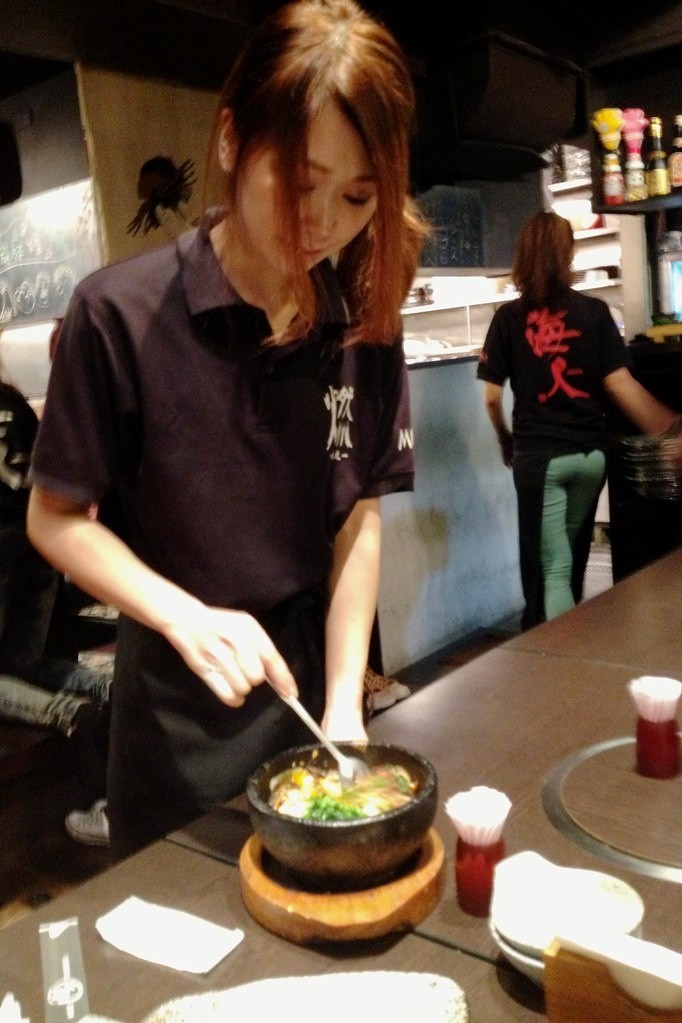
[487,851,645,985]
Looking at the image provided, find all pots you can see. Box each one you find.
[246,739,438,879]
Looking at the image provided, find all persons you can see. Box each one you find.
[0,0,447,864]
[477,213,682,632]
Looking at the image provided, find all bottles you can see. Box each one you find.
[603,151,626,206]
[665,114,682,195]
[647,116,671,199]
[625,146,649,202]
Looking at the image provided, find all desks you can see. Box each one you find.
[0,544,682,1022]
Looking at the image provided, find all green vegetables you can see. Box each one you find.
[309,798,363,820]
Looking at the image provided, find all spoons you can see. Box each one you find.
[265,676,370,784]
